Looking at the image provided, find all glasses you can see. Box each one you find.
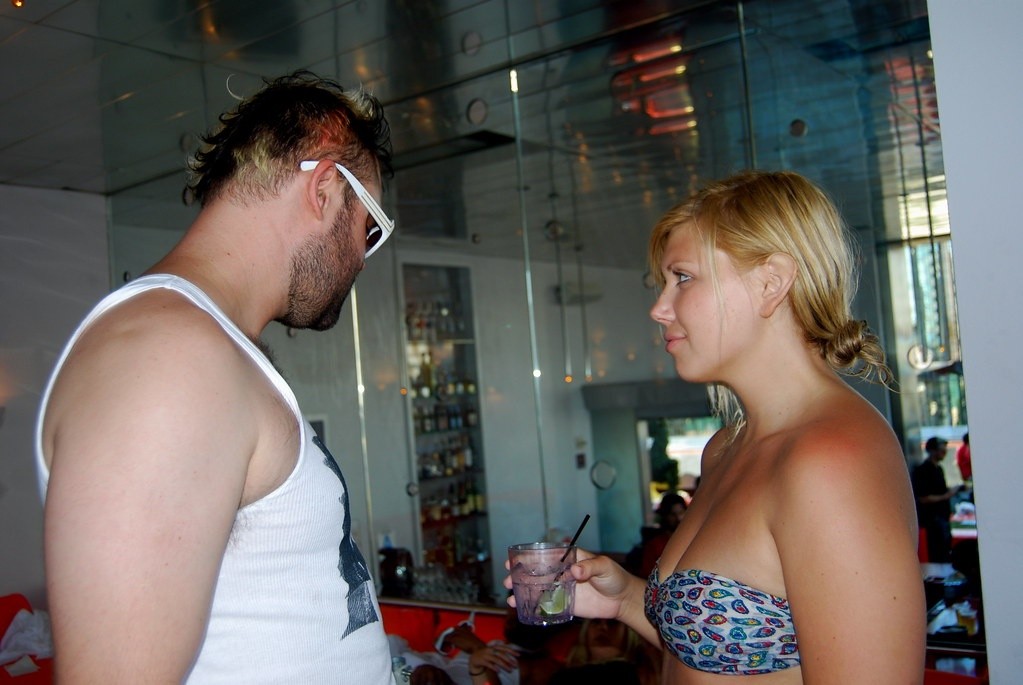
[300,160,395,258]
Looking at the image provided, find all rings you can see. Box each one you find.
[493,648,498,655]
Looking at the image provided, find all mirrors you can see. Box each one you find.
[91,1,984,609]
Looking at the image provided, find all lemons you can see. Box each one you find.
[541,588,571,616]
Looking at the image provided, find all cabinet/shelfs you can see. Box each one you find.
[406,337,492,571]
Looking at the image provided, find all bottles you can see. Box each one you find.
[405,299,488,573]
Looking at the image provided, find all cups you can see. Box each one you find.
[953,604,978,636]
[507,542,577,625]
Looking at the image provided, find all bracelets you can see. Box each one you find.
[469,667,487,676]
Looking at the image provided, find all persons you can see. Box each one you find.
[912,437,960,563]
[956,432,973,490]
[34,66,399,685]
[388,494,689,685]
[504,171,927,685]
